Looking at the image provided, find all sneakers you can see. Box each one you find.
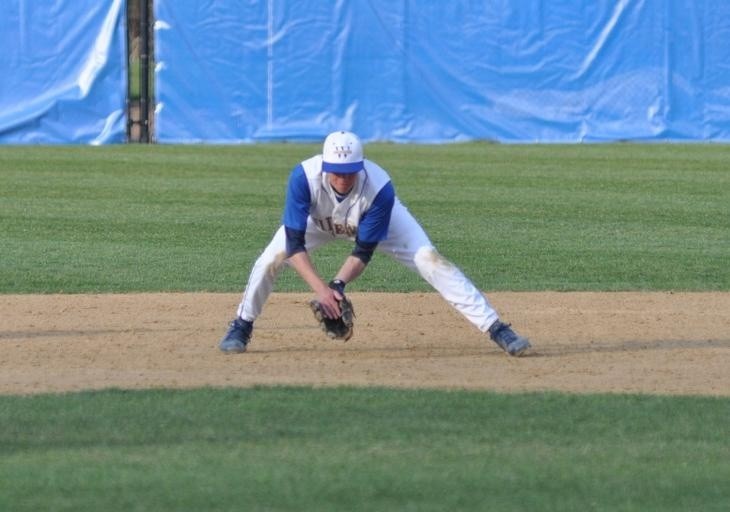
[220,319,252,352]
[490,323,531,357]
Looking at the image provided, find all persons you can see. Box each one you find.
[216,129,532,358]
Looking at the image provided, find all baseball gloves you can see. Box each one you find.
[310,280,355,342]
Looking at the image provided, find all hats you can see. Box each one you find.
[322,131,363,173]
